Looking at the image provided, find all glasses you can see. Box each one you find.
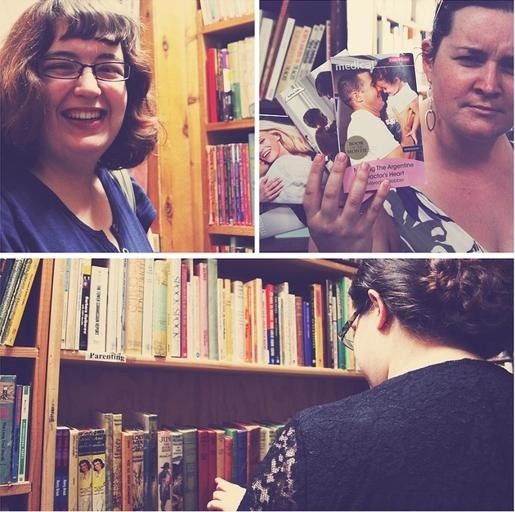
[37,55,133,83]
[336,310,359,351]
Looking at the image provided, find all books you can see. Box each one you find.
[243,283,253,362]
[307,282,324,371]
[17,385,30,483]
[257,277,263,364]
[215,428,224,481]
[170,258,183,357]
[5,256,39,351]
[273,286,280,364]
[224,278,232,363]
[265,283,274,365]
[2,257,31,349]
[178,262,189,358]
[0,372,15,485]
[297,24,318,76]
[141,430,151,512]
[183,258,196,358]
[289,293,298,366]
[93,409,113,512]
[290,25,310,88]
[118,258,126,355]
[82,256,91,353]
[198,429,209,512]
[267,430,274,450]
[301,304,313,367]
[151,260,168,357]
[120,433,134,512]
[103,427,114,512]
[207,430,219,505]
[181,426,197,512]
[87,266,108,357]
[1,256,24,348]
[232,282,246,363]
[216,275,226,362]
[195,262,209,358]
[156,428,173,512]
[187,278,192,359]
[11,382,24,484]
[190,272,200,360]
[61,428,70,512]
[266,16,296,101]
[312,22,328,67]
[275,423,283,439]
[129,429,144,512]
[223,435,232,483]
[251,285,260,364]
[254,9,273,24]
[258,18,273,84]
[262,287,268,364]
[334,285,345,368]
[166,261,171,359]
[102,258,122,360]
[260,427,267,461]
[209,237,253,255]
[65,259,78,351]
[171,431,184,512]
[126,259,145,357]
[273,49,344,161]
[276,290,286,364]
[54,423,62,512]
[348,280,356,370]
[243,422,256,488]
[258,113,321,239]
[235,429,246,488]
[133,408,157,512]
[331,283,338,369]
[278,280,290,365]
[0,253,26,335]
[76,258,82,353]
[277,22,302,98]
[92,427,108,512]
[112,412,123,511]
[342,277,349,369]
[211,423,240,486]
[203,131,255,229]
[142,258,154,359]
[69,430,79,512]
[0,258,15,305]
[252,424,260,483]
[201,34,257,120]
[329,49,428,194]
[197,0,256,26]
[324,17,332,61]
[77,425,92,512]
[204,260,220,360]
[295,296,305,368]
[61,257,71,353]
[319,278,333,368]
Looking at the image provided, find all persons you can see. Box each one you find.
[199,252,514,512]
[315,69,338,106]
[92,457,106,487]
[0,0,165,256]
[303,105,340,158]
[373,65,425,163]
[256,127,313,211]
[300,0,514,253]
[339,71,404,165]
[79,459,92,487]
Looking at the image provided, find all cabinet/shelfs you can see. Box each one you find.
[258,0,348,253]
[194,1,255,254]
[0,258,54,512]
[40,260,371,510]
